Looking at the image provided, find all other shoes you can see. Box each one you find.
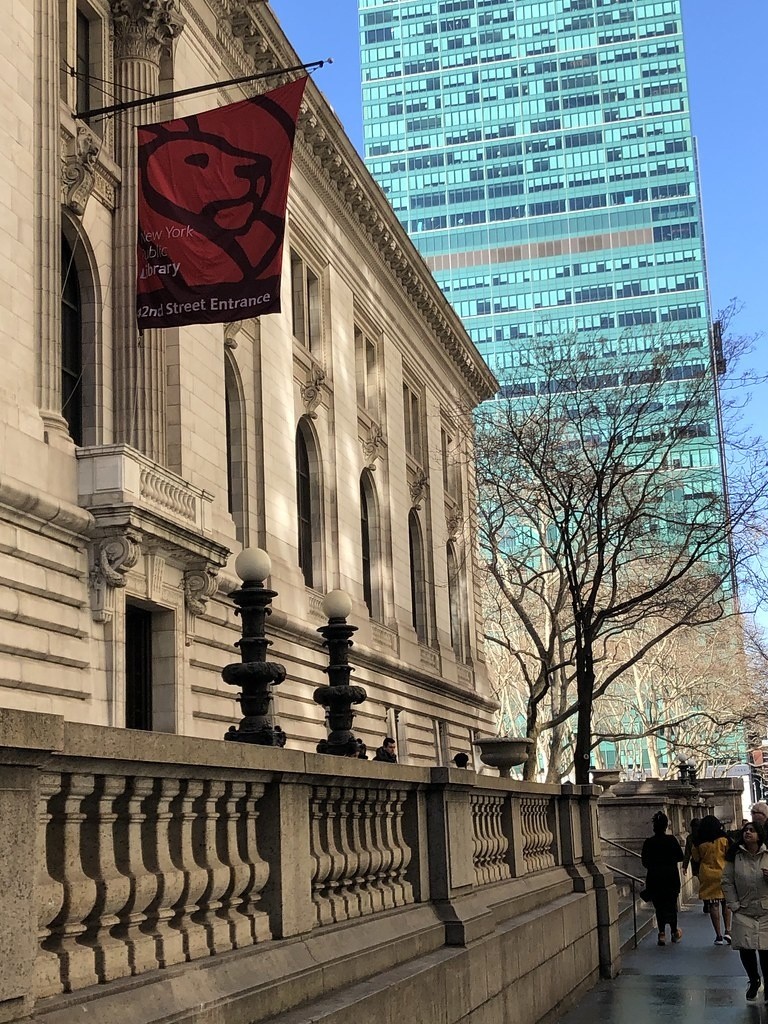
[658,932,666,946]
[764,990,768,1005]
[746,977,761,1004]
[671,928,682,942]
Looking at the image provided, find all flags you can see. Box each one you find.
[136,75,308,330]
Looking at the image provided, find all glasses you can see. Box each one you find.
[743,828,755,833]
[751,810,764,815]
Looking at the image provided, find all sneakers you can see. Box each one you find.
[724,933,732,943]
[714,936,723,945]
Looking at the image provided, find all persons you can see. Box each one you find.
[640,811,685,946]
[682,801,768,913]
[721,822,768,1002]
[698,815,732,944]
